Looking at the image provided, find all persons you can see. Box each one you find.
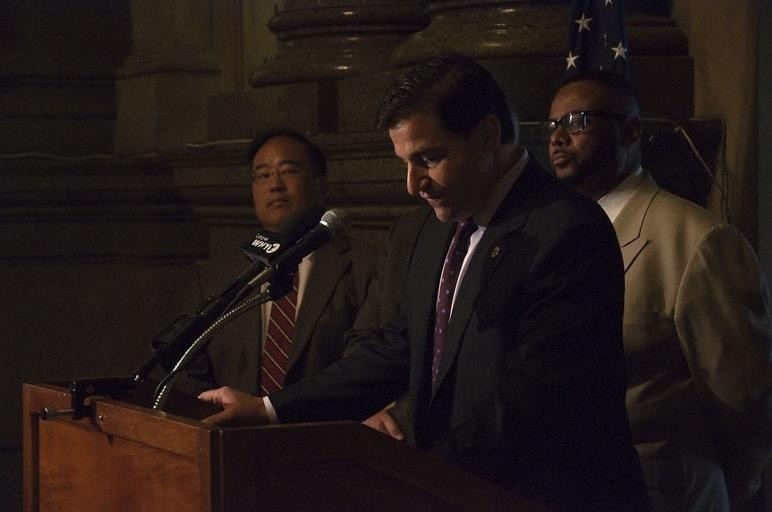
[535,72,772,512]
[205,127,414,440]
[197,54,651,512]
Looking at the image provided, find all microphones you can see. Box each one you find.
[237,209,349,299]
[151,208,326,376]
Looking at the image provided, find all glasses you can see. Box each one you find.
[538,109,628,140]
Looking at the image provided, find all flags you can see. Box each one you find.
[557,0,628,87]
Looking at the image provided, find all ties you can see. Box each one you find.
[426,220,478,398]
[256,265,299,397]
